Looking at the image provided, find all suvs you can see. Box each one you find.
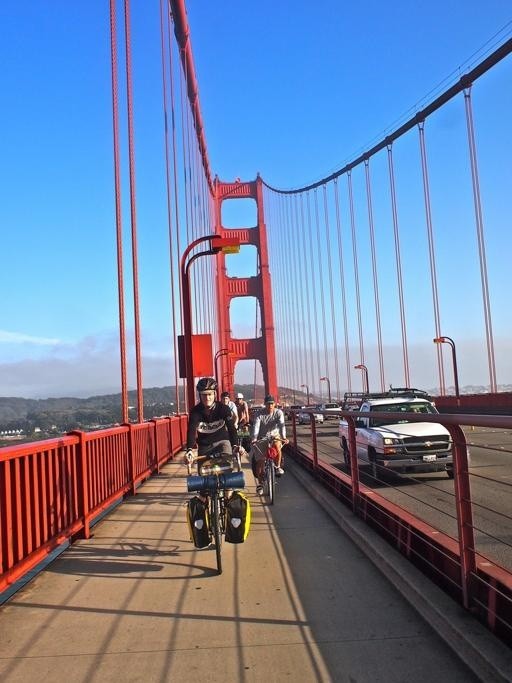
[339,388,453,484]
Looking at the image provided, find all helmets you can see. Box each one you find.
[196,378,218,390]
[234,393,244,399]
[221,392,230,398]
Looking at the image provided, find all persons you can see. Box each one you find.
[182,376,244,516]
[218,390,239,430]
[233,391,250,423]
[248,394,287,495]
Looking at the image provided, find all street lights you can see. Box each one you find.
[320,376,331,402]
[182,235,240,416]
[355,365,369,394]
[434,337,459,402]
[301,384,309,405]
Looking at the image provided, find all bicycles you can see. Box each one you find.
[187,422,288,574]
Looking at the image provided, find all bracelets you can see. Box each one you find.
[186,448,192,453]
[232,445,236,450]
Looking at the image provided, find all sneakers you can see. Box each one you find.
[257,484,264,496]
[275,466,285,475]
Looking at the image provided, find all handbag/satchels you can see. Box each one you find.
[186,497,213,552]
[223,490,251,545]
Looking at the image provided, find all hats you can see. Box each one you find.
[265,395,275,404]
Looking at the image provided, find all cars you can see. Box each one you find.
[287,403,341,424]
